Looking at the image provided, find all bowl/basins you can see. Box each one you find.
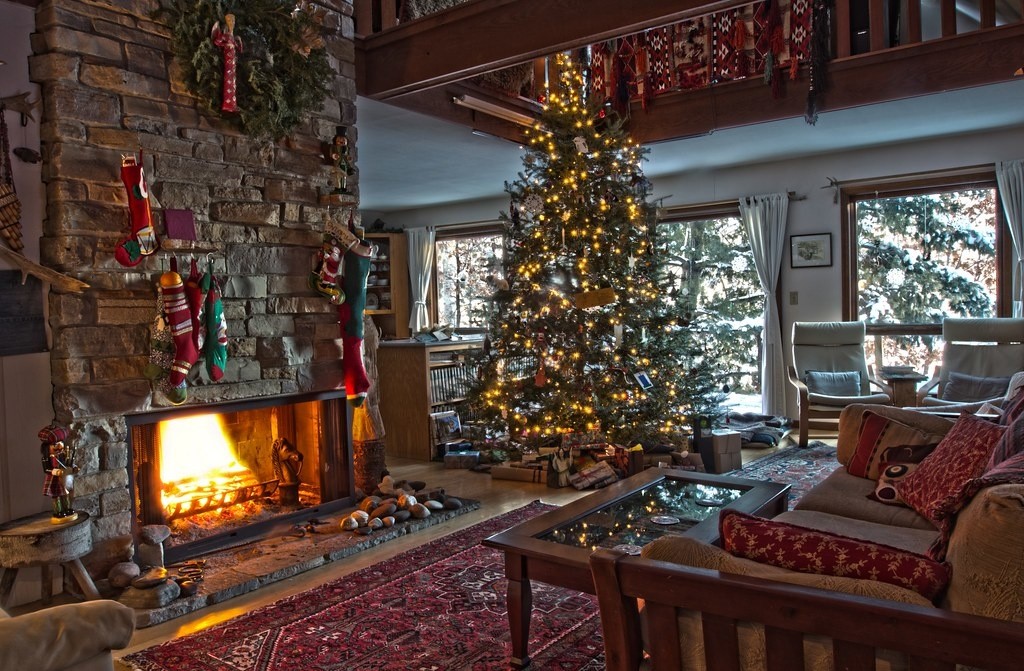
[378,252,388,259]
[377,279,389,286]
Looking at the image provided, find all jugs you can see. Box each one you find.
[368,240,380,261]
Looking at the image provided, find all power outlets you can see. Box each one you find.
[789,291,799,305]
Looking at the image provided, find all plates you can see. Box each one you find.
[366,292,380,310]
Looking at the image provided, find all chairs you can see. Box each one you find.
[916,317,1023,424]
[782,319,891,450]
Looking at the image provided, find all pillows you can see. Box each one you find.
[862,461,919,509]
[842,409,946,482]
[716,506,954,603]
[941,370,1011,412]
[894,410,1010,534]
[803,368,864,401]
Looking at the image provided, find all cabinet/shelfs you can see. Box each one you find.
[358,229,510,464]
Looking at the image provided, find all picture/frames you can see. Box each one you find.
[788,232,833,271]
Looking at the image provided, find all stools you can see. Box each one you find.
[0,509,105,618]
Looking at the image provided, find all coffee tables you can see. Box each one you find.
[479,463,793,671]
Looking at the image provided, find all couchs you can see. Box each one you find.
[580,370,1024,670]
[0,597,134,670]
